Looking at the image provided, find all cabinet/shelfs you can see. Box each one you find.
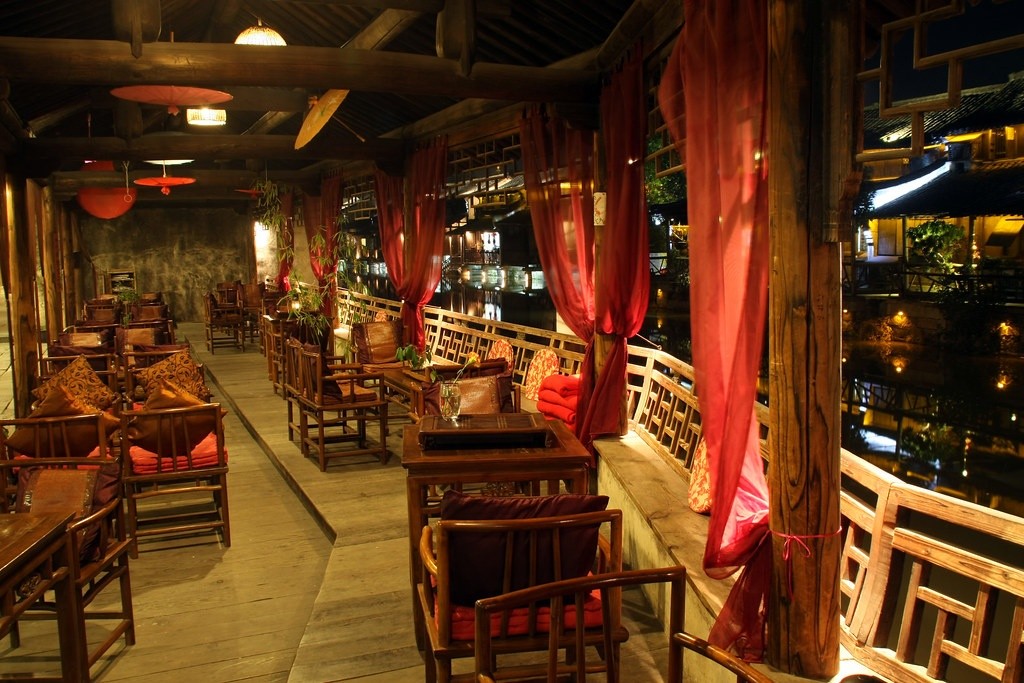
[107,268,137,293]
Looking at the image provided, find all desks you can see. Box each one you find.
[263,314,335,380]
[404,419,591,651]
[0,510,80,683]
[382,366,421,440]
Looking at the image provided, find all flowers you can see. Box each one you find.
[394,344,482,411]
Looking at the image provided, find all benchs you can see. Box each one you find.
[430,356,892,683]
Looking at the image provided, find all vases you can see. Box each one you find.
[440,383,463,420]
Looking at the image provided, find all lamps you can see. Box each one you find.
[186,109,227,126]
[235,17,288,46]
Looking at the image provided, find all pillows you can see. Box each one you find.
[444,487,609,609]
[352,317,403,364]
[62,341,107,372]
[133,348,215,399]
[3,382,122,458]
[115,327,159,364]
[525,349,559,402]
[429,373,515,414]
[30,353,117,410]
[374,309,389,321]
[118,377,229,457]
[689,432,714,514]
[14,465,120,567]
[304,340,344,397]
[59,328,111,348]
[133,344,191,368]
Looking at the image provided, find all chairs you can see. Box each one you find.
[1,291,231,683]
[410,363,776,683]
[204,281,520,473]
